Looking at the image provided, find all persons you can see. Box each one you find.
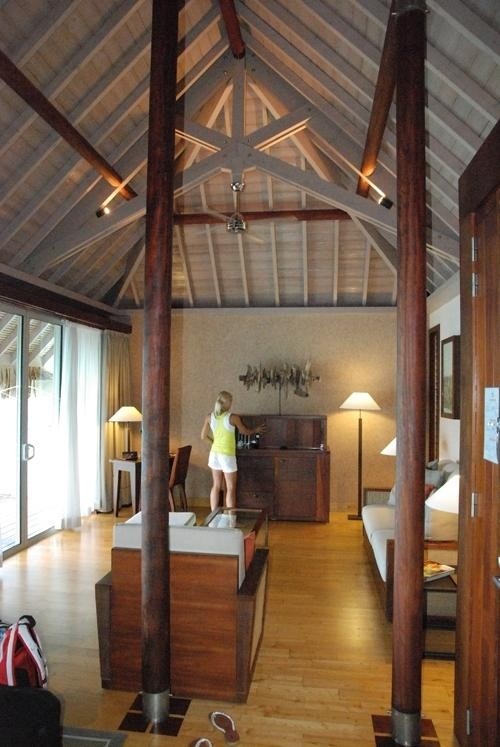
[201,391,267,527]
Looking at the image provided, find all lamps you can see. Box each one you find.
[338,390,381,520]
[381,437,397,457]
[96,206,110,218]
[107,406,143,459]
[378,195,394,209]
[425,475,460,588]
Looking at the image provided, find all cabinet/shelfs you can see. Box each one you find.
[220,412,330,523]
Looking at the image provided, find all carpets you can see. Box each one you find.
[63,725,127,746]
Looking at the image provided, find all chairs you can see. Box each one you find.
[166,445,191,509]
[94,522,272,704]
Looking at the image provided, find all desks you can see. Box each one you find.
[109,455,141,516]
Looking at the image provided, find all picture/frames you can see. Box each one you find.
[440,335,459,419]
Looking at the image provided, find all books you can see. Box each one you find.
[424,559,455,582]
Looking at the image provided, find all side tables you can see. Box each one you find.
[422,563,457,661]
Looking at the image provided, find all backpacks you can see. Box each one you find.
[0,613,49,689]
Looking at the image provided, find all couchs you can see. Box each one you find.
[362,460,462,616]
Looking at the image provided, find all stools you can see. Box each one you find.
[125,510,196,526]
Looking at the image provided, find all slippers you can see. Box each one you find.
[191,737,213,747]
[209,711,240,745]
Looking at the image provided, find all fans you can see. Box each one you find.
[190,175,297,245]
